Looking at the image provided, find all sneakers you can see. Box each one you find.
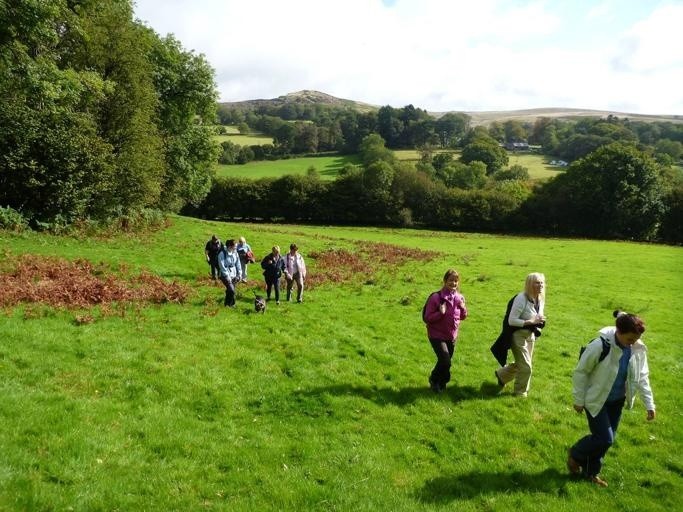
[567,447,609,488]
[495,370,527,398]
[429,377,447,391]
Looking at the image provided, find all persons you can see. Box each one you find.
[236,236,255,283]
[283,243,307,302]
[566,307,657,487]
[260,245,292,305]
[422,268,468,393]
[217,238,243,309]
[205,234,225,280]
[494,272,547,398]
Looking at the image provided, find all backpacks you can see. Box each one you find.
[578,336,610,362]
[423,291,443,324]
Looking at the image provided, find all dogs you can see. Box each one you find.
[254,295,265,314]
